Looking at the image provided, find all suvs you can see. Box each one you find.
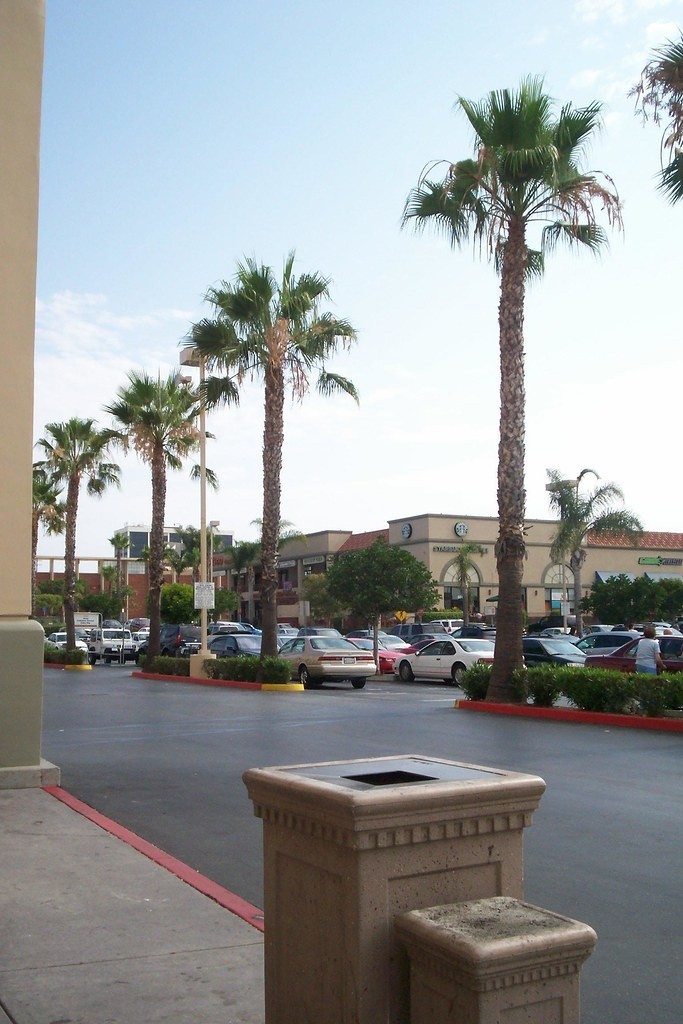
[59,626,91,647]
[134,623,202,665]
[88,626,137,665]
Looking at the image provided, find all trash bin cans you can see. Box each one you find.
[242,755,545,1024]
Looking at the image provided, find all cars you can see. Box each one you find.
[277,634,378,691]
[519,636,587,668]
[400,632,456,646]
[46,631,89,658]
[568,631,642,657]
[583,634,683,674]
[101,617,346,654]
[345,630,388,641]
[395,638,445,654]
[391,638,528,689]
[207,633,285,661]
[386,614,683,646]
[376,634,412,650]
[344,636,399,674]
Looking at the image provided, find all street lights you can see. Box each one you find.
[545,478,577,633]
[180,347,208,654]
[209,520,221,582]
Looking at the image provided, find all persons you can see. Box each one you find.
[673,624,680,631]
[474,611,482,622]
[663,629,673,635]
[628,623,638,632]
[587,627,592,635]
[635,627,667,708]
[568,626,578,637]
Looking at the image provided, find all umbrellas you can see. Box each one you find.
[486,595,499,601]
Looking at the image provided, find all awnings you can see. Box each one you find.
[595,571,637,584]
[645,572,683,582]
